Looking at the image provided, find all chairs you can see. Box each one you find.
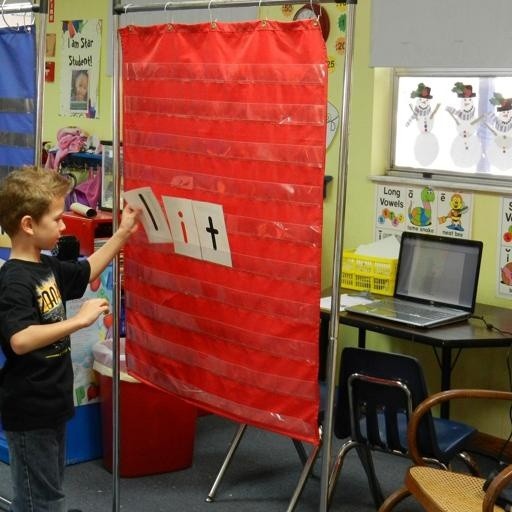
[324,347,512,512]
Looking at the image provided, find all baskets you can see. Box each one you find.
[340,247,398,297]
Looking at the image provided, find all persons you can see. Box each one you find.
[69,70,89,110]
[0,161,142,512]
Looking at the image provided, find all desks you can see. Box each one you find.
[318,284,511,512]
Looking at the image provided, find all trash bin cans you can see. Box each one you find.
[92,338,197,477]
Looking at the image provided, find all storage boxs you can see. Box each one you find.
[92,360,200,479]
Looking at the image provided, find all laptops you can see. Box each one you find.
[343,231,484,331]
